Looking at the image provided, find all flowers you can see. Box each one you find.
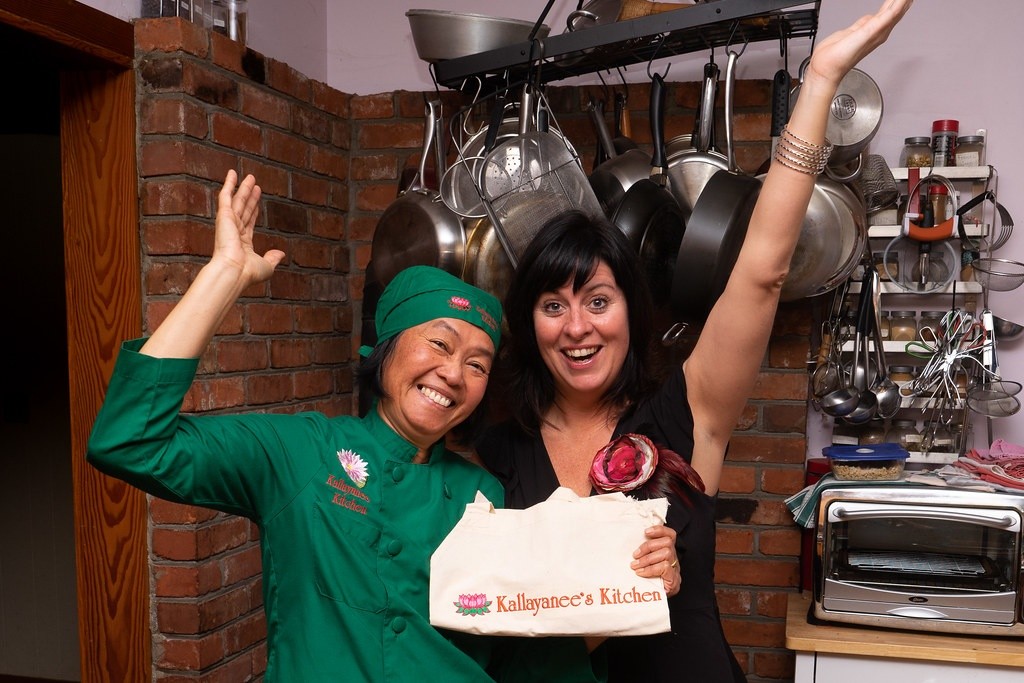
[589,432,660,496]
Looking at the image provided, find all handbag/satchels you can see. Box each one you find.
[430,492,669,636]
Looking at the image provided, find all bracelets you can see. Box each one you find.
[773,123,834,176]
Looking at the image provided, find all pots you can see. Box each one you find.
[361,42,883,345]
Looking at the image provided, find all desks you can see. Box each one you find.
[783,590,1024,683]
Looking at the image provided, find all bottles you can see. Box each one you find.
[193,0,203,26]
[140,0,177,18]
[178,1,191,21]
[228,0,251,47]
[203,0,212,30]
[899,135,934,167]
[952,135,985,167]
[931,118,958,168]
[211,1,229,37]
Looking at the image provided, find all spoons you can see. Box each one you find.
[804,152,1023,426]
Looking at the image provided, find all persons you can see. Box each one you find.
[450,0,912,682]
[85,167,684,682]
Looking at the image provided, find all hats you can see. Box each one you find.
[376,266,503,355]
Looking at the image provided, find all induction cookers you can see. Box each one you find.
[803,471,1023,642]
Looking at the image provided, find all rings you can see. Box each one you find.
[671,559,677,568]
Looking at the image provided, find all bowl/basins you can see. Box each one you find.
[820,442,910,483]
[404,4,553,64]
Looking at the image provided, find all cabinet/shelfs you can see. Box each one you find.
[823,162,999,467]
